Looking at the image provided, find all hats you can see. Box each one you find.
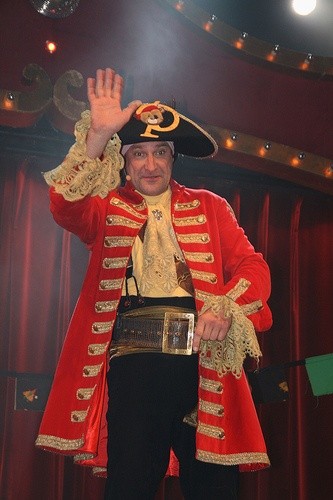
[117,101,217,160]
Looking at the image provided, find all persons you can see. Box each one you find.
[35,68,272,500]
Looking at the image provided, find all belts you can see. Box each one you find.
[112,313,194,355]
